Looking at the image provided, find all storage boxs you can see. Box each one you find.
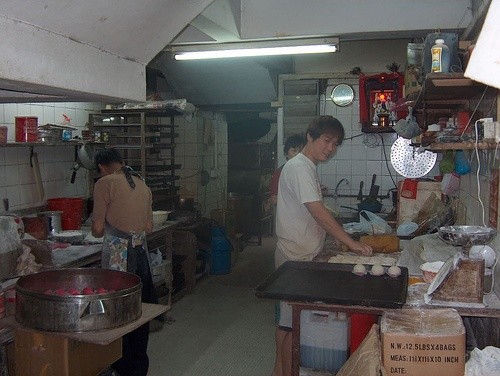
[382,309,465,376]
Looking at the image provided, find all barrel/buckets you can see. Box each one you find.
[15,115,38,142]
[300,310,351,371]
[210,226,233,276]
[47,198,84,231]
[22,211,64,240]
[348,313,378,357]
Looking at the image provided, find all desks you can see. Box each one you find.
[0,221,180,376]
[288,214,500,376]
[171,219,213,293]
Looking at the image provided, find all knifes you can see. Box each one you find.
[369,175,380,201]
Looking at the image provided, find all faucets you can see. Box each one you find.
[333,178,350,196]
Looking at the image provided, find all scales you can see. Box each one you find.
[426,226,496,302]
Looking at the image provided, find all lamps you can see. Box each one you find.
[169,35,339,60]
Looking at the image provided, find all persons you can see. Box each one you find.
[273,135,308,322]
[271,115,373,376]
[91,150,165,376]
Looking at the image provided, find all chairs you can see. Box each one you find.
[234,188,274,247]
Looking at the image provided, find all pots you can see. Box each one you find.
[201,155,209,185]
[340,203,383,214]
[78,135,95,170]
[15,268,143,334]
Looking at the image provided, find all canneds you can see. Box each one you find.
[95,131,109,142]
[0,126,8,144]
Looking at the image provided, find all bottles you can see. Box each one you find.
[62,119,73,141]
[0,215,24,282]
[95,130,111,143]
[0,127,8,144]
[430,38,450,72]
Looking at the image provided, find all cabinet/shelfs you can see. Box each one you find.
[405,73,496,151]
[87,111,183,218]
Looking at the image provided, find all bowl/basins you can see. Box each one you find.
[419,261,444,283]
[177,195,194,210]
[153,211,172,228]
